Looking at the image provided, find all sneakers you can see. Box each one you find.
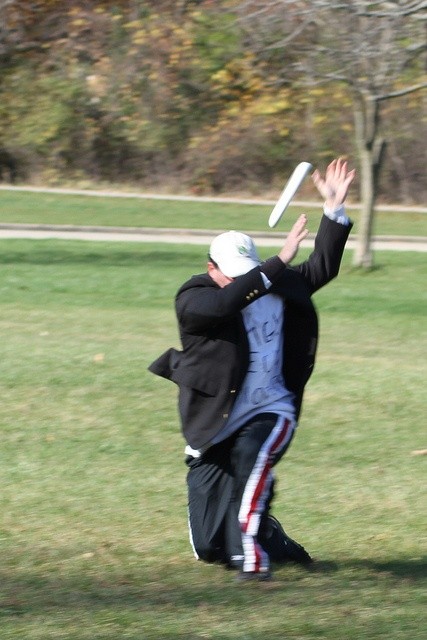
[265,514,313,564]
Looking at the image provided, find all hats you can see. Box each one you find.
[210,229,262,279]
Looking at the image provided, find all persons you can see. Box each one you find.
[145,156,357,582]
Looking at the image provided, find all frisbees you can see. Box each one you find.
[268,160,313,228]
